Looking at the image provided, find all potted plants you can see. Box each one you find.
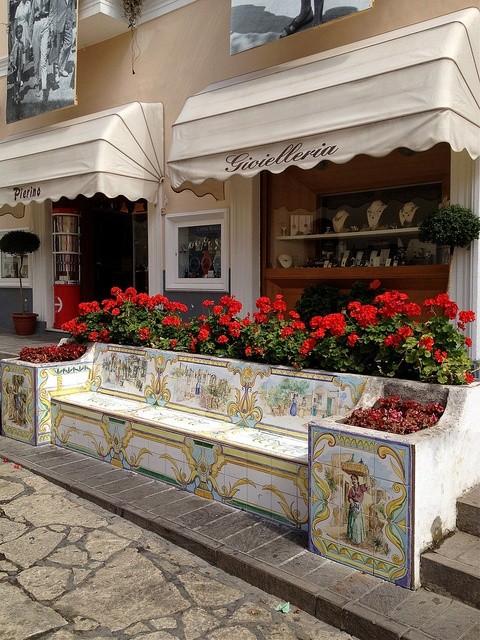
[418,204,480,275]
[0,231,39,336]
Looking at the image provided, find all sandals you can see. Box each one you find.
[279,7,315,38]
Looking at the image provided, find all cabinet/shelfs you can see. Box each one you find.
[260,159,448,319]
[52,210,84,284]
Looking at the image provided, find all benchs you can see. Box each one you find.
[51,340,364,536]
